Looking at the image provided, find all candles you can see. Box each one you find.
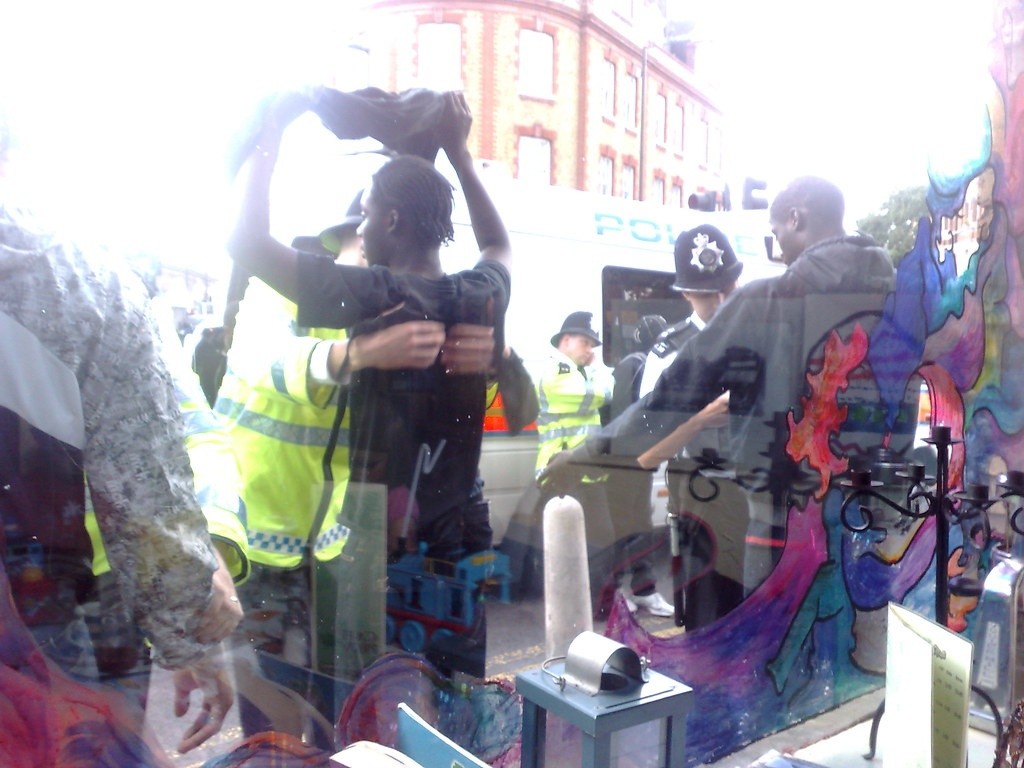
[933,423,951,442]
[967,482,988,499]
[908,463,925,477]
[1007,470,1024,484]
[702,448,718,459]
[851,468,872,484]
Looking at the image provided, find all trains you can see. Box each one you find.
[385,540,514,655]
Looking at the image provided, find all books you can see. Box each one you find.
[395,702,495,768]
[328,740,424,768]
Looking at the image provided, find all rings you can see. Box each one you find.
[230,596,239,604]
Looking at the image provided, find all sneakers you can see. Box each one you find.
[626,598,638,613]
[633,593,676,617]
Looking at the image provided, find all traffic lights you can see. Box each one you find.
[740,174,770,210]
[687,183,717,213]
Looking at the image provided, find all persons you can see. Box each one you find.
[480,341,541,434]
[1,206,234,768]
[147,91,514,760]
[535,170,924,637]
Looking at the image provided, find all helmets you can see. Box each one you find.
[319,188,364,254]
[551,312,603,349]
[671,224,743,293]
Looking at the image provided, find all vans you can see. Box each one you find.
[439,181,775,558]
